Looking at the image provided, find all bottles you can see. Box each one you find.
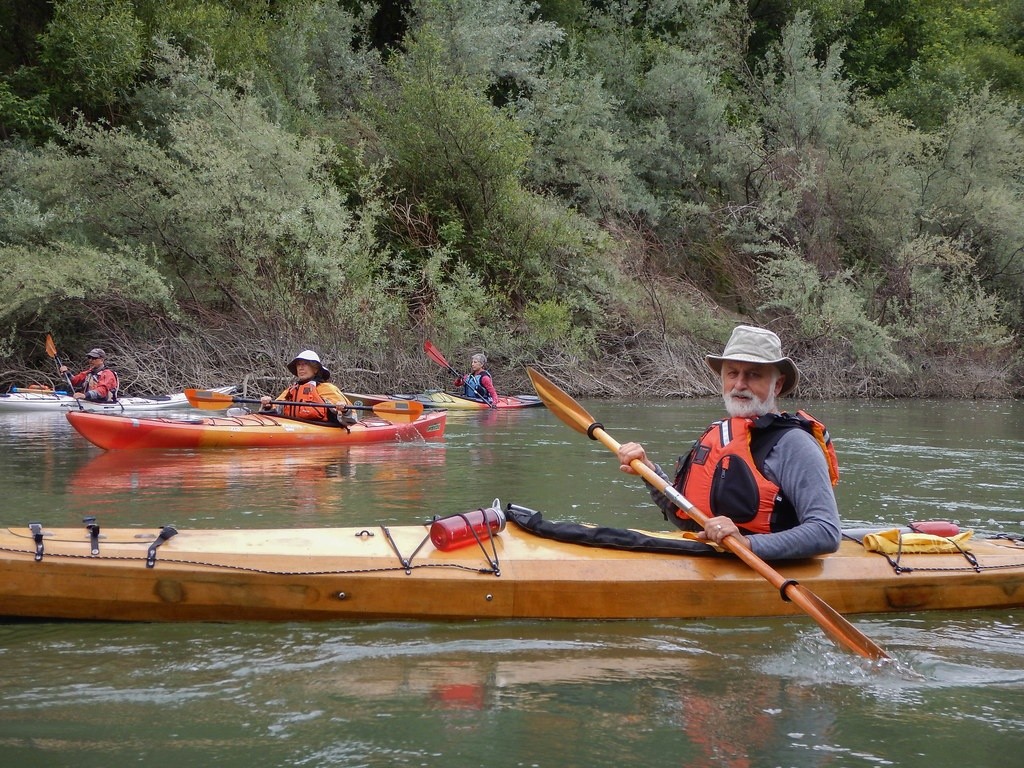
[425,389,441,395]
[28,384,49,389]
[226,407,252,417]
[354,400,364,417]
[430,498,506,552]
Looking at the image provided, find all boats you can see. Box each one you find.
[0,385,237,412]
[343,392,543,410]
[0,497,1023,619]
[66,409,446,450]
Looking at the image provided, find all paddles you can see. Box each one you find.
[45,333,85,411]
[182,387,424,424]
[423,340,497,409]
[526,366,894,659]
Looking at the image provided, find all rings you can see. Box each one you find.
[717,525,722,529]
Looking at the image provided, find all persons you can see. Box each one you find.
[454,354,500,404]
[259,349,357,425]
[60,348,117,402]
[618,326,841,562]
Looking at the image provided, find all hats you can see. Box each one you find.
[85,348,106,358]
[705,325,800,399]
[287,350,331,384]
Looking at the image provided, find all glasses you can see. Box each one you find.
[87,356,101,360]
